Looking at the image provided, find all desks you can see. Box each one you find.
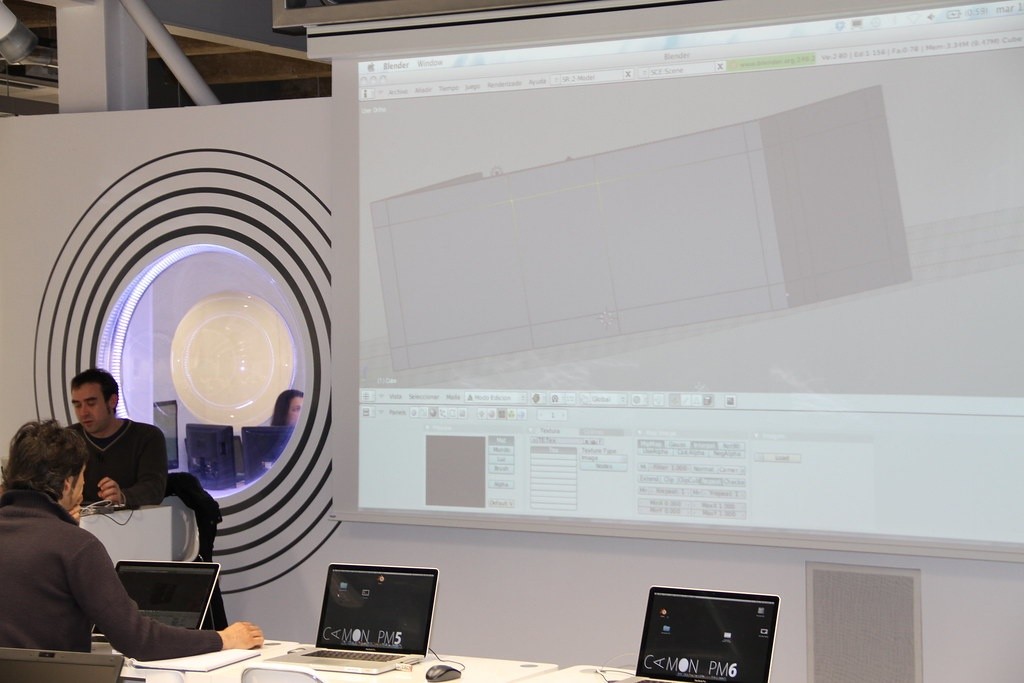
[89,632,643,683]
[77,503,190,570]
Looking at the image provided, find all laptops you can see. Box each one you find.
[613,585,781,683]
[260,563,439,674]
[88,560,221,654]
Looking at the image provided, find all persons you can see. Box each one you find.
[270,390,305,425]
[0,421,263,662]
[65,369,168,509]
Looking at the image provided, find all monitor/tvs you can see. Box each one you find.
[239,425,295,482]
[151,399,180,470]
[185,423,237,490]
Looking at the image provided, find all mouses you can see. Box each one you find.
[425,665,462,683]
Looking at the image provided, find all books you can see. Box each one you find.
[131,647,261,672]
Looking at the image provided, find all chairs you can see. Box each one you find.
[160,474,219,632]
[0,646,124,683]
